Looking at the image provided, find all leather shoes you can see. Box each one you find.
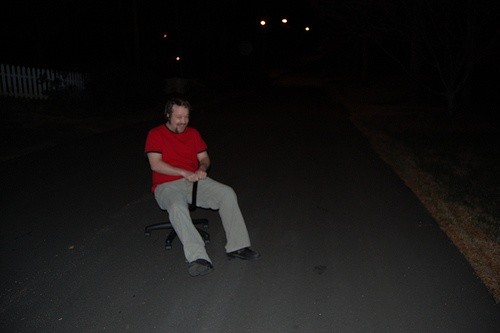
[189,258,214,277]
[227,247,262,261]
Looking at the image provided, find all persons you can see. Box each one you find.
[144,96,263,277]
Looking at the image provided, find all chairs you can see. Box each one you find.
[144,178,213,249]
